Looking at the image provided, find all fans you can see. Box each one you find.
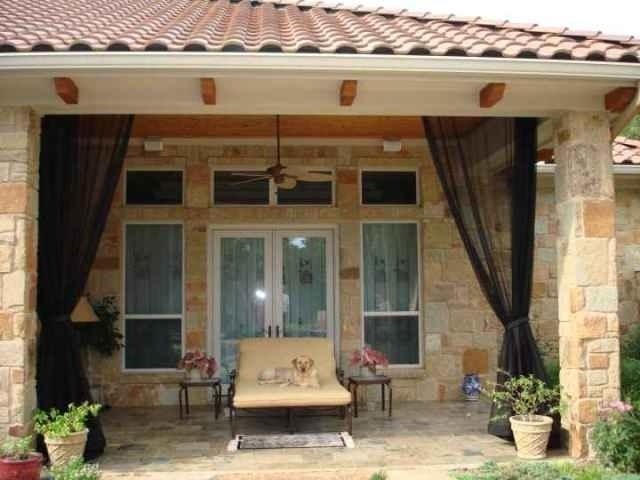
[231,115,334,190]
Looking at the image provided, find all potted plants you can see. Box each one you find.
[33,400,103,467]
[0,435,44,480]
[476,363,572,460]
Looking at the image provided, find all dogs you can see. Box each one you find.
[256,354,321,389]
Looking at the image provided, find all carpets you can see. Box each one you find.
[236,431,344,449]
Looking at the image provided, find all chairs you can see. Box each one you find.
[225,336,352,434]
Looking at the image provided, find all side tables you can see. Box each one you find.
[178,379,222,419]
[348,375,393,418]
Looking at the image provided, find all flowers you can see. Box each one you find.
[176,348,216,378]
[347,343,389,372]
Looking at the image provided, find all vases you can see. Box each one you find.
[361,363,376,377]
[464,374,480,402]
[188,368,201,381]
